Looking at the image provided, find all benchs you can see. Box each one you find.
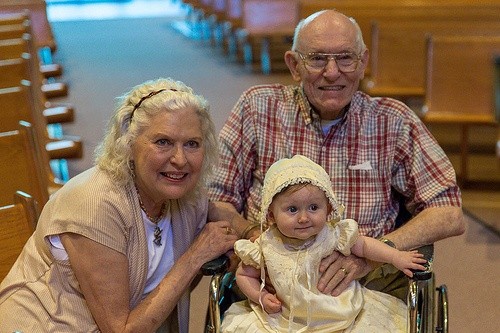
[0,0,84,287]
[174,0,500,191]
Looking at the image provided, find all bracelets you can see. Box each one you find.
[241,222,266,239]
[375,236,397,249]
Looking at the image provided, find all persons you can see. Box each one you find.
[216,154,428,333]
[0,77,241,333]
[206,8,466,307]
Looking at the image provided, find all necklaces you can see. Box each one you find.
[129,155,168,246]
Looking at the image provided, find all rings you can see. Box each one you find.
[226,226,233,235]
[340,266,350,275]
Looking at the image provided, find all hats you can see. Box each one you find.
[260,155,345,226]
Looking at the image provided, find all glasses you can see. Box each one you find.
[296,50,364,73]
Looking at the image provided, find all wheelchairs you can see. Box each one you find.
[194,199,452,333]
